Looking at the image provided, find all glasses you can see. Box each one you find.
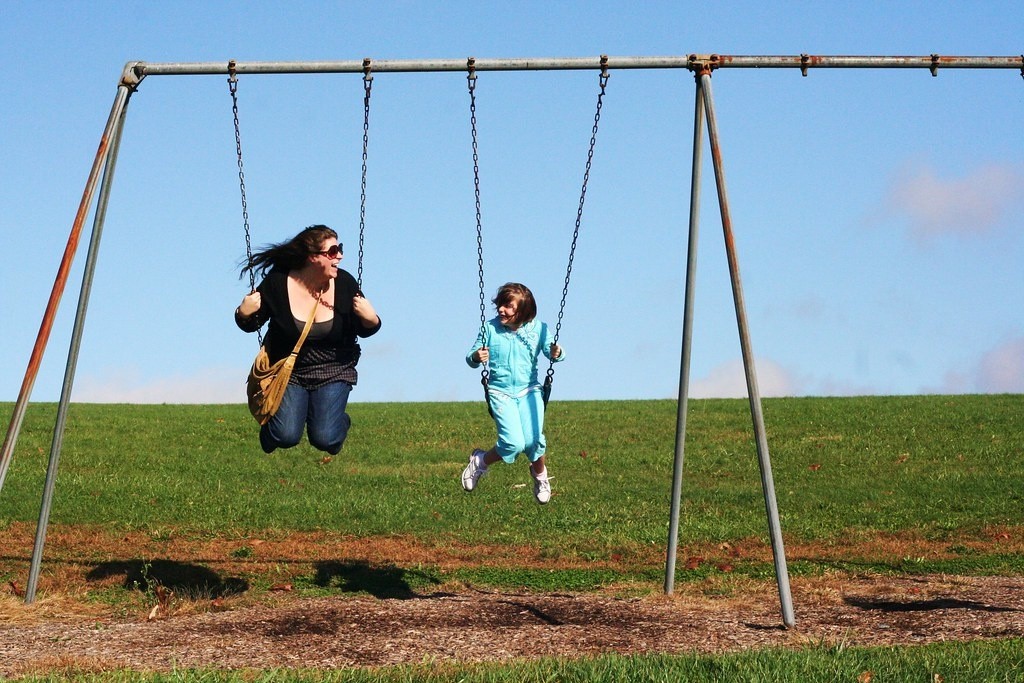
[309,242,344,259]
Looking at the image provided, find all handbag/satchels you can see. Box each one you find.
[245,288,324,424]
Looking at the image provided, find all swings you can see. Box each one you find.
[467,76,612,418]
[228,74,373,366]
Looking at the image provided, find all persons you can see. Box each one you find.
[234,224,382,455]
[461,282,565,504]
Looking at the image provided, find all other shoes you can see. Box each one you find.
[328,411,352,456]
[259,422,280,454]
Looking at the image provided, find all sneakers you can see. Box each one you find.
[529,463,556,505]
[461,448,487,492]
